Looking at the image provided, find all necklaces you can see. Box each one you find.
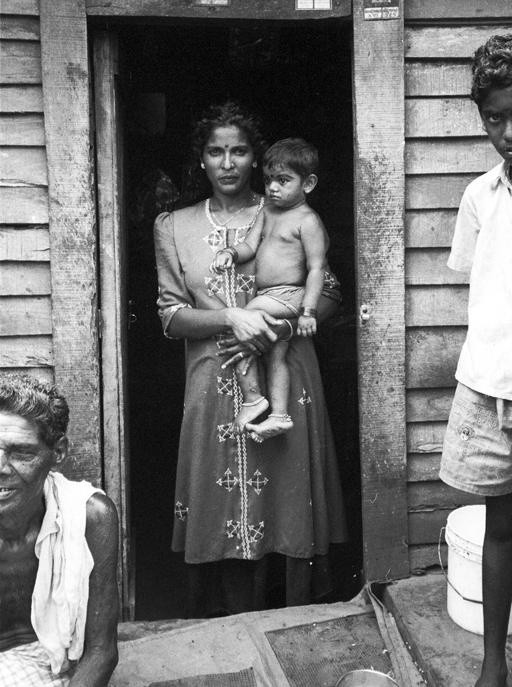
[210,192,257,228]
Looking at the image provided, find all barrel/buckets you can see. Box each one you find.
[335,668,400,687]
[437,504,512,636]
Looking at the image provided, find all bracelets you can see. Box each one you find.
[300,306,317,317]
[215,245,237,264]
[279,315,295,343]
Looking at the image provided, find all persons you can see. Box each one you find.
[438,34,512,686]
[152,91,343,620]
[207,137,330,445]
[0,373,118,687]
